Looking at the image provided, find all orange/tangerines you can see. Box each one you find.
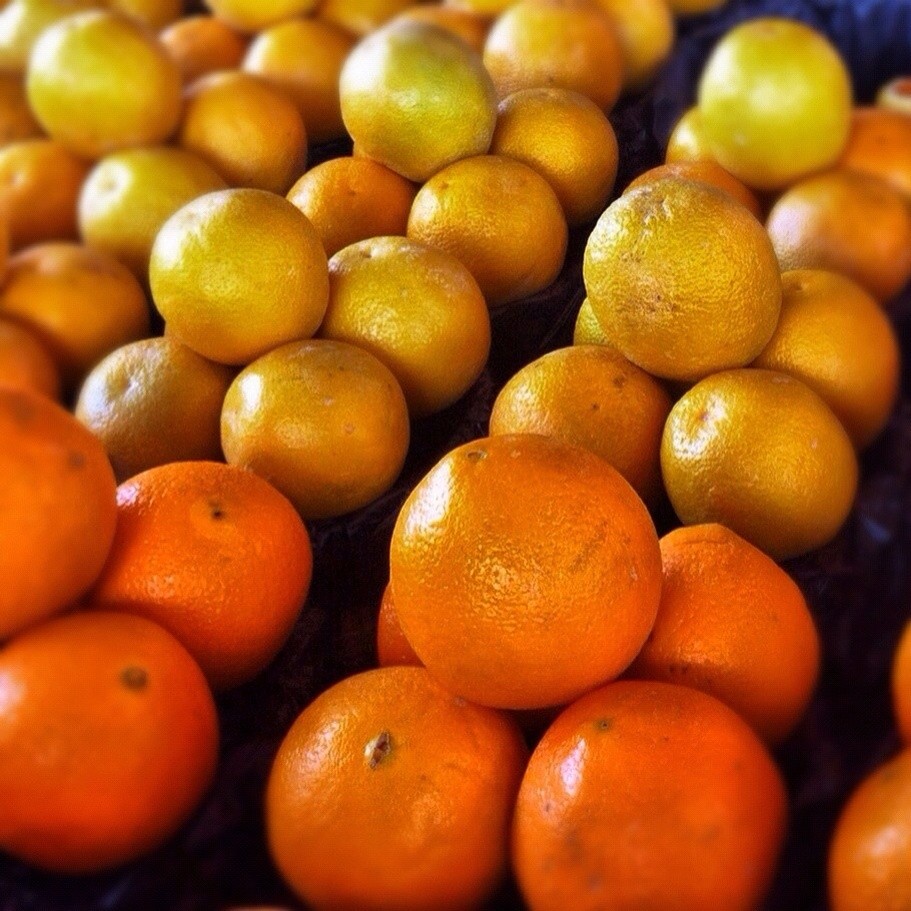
[0,0,728,872]
[266,19,911,911]
